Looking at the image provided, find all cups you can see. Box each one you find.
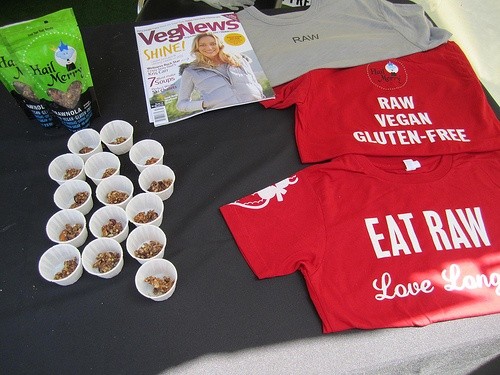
[53,180,93,216]
[48,154,86,186]
[67,129,103,163]
[96,175,134,210]
[84,152,120,185]
[126,224,167,265]
[45,209,88,248]
[38,243,83,286]
[99,120,133,155]
[135,259,177,301]
[82,237,124,279]
[138,164,175,201]
[129,139,164,173]
[89,205,129,243]
[126,193,164,227]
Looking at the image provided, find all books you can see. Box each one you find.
[134,12,276,127]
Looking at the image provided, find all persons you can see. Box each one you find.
[176,32,267,111]
